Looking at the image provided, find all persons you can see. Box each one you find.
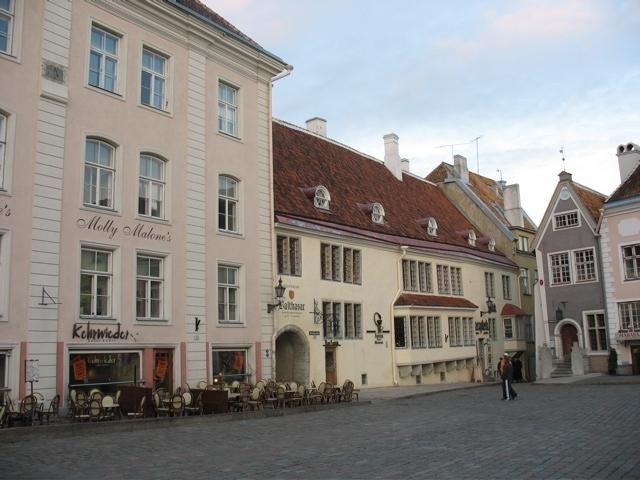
[497,352,522,401]
[608,348,618,375]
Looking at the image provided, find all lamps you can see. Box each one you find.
[480,297,493,317]
[267,275,286,313]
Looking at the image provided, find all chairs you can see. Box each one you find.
[151,383,203,422]
[127,395,147,424]
[198,379,354,416]
[12,393,60,427]
[64,388,124,424]
